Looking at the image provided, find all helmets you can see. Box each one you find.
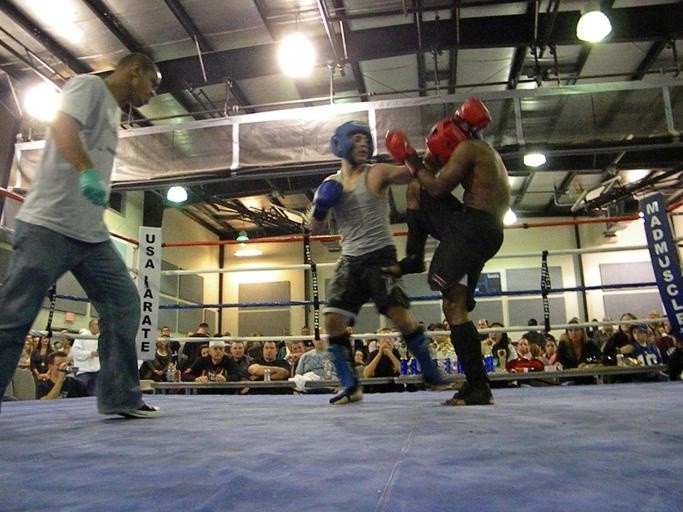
[425,116,473,165]
[330,120,373,156]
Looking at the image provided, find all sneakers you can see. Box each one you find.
[127,403,161,418]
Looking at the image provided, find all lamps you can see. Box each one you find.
[236,217,249,240]
[167,186,188,203]
[576,9,612,43]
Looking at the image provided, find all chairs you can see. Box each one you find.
[3,367,37,401]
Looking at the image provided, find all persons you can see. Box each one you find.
[0,51,163,418]
[138,319,456,395]
[19,318,101,400]
[384,96,512,405]
[305,119,457,405]
[476,314,683,387]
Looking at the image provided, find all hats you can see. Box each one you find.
[209,340,231,348]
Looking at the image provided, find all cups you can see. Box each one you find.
[398,353,494,376]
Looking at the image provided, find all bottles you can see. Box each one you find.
[166,361,175,383]
[615,347,624,368]
[263,368,271,382]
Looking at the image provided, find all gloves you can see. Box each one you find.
[79,170,110,206]
[453,96,492,134]
[384,129,426,177]
[313,180,343,221]
